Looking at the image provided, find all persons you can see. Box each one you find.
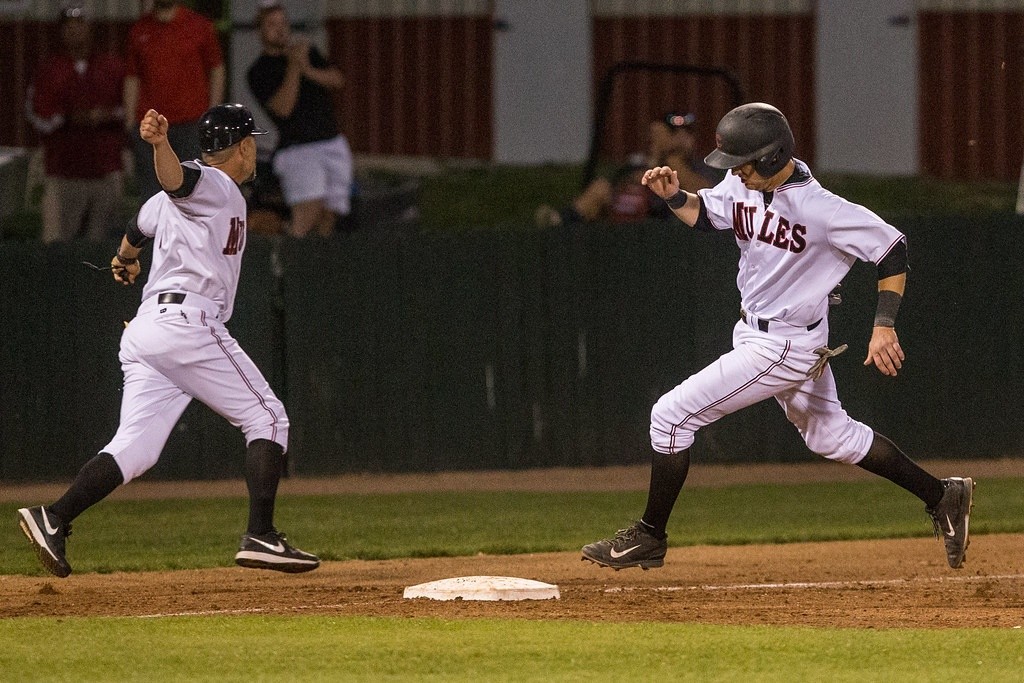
[17,103,321,577]
[25,0,355,244]
[581,103,977,572]
[533,107,712,226]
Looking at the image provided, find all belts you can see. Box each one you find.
[158,294,188,305]
[739,310,823,334]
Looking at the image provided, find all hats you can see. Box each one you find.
[651,96,694,130]
[255,0,283,21]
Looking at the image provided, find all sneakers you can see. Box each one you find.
[17,505,73,578]
[579,521,668,571]
[926,476,976,569]
[235,530,318,571]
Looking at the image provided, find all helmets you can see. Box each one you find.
[701,102,796,179]
[198,103,268,152]
